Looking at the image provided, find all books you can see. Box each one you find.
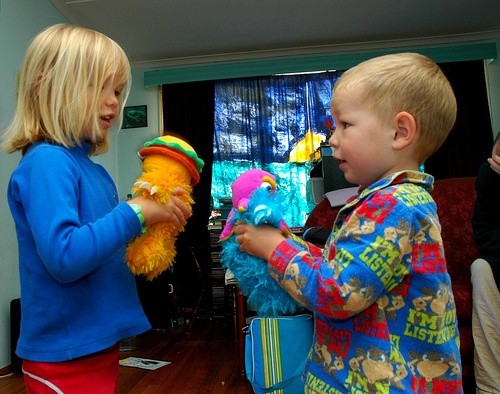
[211,251,220,262]
[210,231,222,248]
[119,357,172,370]
[212,283,226,305]
[212,264,226,279]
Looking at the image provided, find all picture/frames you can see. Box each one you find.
[122,105,147,129]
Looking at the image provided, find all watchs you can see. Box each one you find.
[126,201,149,234]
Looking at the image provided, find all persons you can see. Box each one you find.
[471,130,500,394]
[233,52,463,394]
[0,22,192,394]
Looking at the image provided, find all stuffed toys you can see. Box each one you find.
[124,135,204,281]
[218,168,315,317]
